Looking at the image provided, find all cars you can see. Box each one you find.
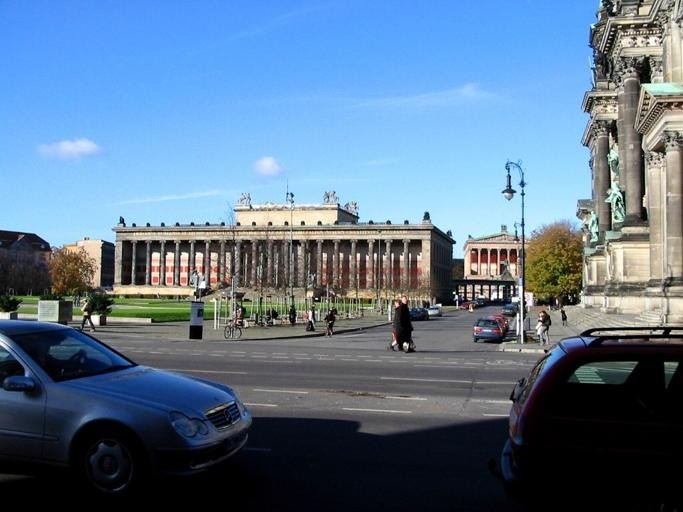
[501,303,518,317]
[460,300,484,310]
[472,315,508,342]
[0,320,253,494]
[501,325,683,484]
[410,305,441,321]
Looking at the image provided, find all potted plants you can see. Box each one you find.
[0,295,22,319]
[86,289,115,326]
[38,294,72,322]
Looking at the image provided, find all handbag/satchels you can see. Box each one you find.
[536,322,547,335]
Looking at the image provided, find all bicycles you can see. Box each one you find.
[223,320,242,339]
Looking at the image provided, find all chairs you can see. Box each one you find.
[29,343,65,381]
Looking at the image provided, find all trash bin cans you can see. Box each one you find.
[101,315,106,326]
[11,313,18,320]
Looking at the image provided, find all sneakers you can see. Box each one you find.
[390,344,416,352]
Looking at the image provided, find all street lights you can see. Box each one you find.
[502,161,525,344]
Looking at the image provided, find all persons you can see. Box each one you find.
[536,312,544,342]
[389,295,416,351]
[79,300,94,332]
[322,309,335,336]
[537,311,551,345]
[287,305,296,326]
[393,298,412,353]
[227,303,243,326]
[305,308,315,331]
[587,211,599,242]
[560,309,567,328]
[605,188,624,221]
